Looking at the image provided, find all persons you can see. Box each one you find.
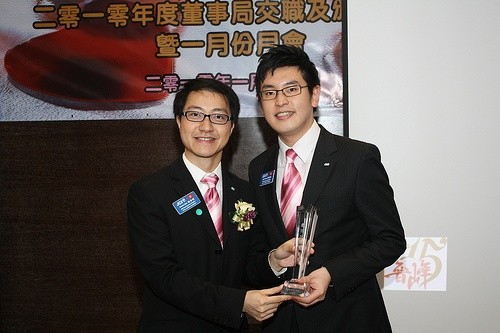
[127,78,314,333]
[248,43,407,333]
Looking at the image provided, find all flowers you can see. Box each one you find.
[228,199,257,231]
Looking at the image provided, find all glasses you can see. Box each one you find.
[259,85,309,100]
[180,111,233,125]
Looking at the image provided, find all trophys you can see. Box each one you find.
[280,206,319,298]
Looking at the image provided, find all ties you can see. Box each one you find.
[200,174,223,249]
[280,149,302,239]
